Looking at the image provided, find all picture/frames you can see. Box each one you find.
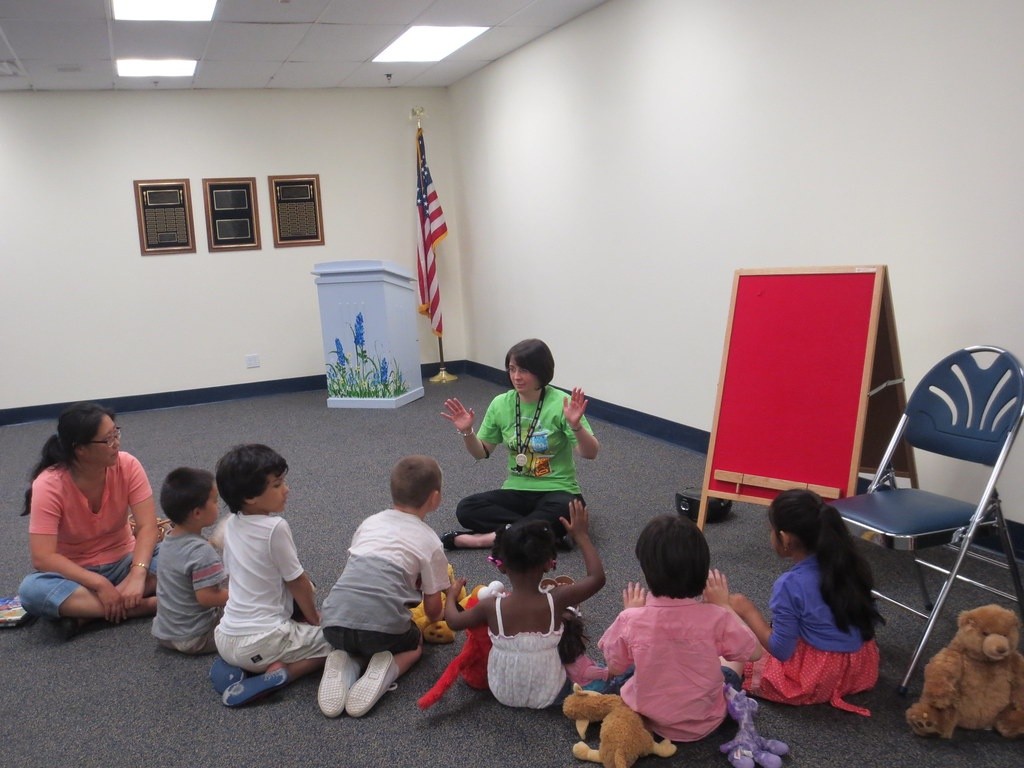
[133,179,197,256]
[267,172,326,248]
[201,176,262,254]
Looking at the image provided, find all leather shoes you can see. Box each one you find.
[440,530,477,551]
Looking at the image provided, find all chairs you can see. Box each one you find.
[819,345,1024,695]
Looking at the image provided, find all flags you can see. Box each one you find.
[416,127,448,340]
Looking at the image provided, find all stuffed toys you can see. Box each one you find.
[410,564,506,708]
[564,683,677,768]
[905,605,1024,736]
[719,685,788,768]
[558,608,635,694]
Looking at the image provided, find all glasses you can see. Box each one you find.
[88,426,122,448]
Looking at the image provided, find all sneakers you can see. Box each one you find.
[317,648,360,718]
[344,649,399,718]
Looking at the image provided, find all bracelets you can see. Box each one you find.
[132,563,146,569]
[572,427,581,431]
[457,428,473,437]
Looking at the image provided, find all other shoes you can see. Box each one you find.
[209,654,249,693]
[221,667,289,708]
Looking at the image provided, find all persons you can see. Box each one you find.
[598,516,761,743]
[209,444,334,708]
[444,498,605,710]
[440,339,599,552]
[729,488,880,717]
[152,467,229,654]
[19,401,160,623]
[318,456,451,718]
